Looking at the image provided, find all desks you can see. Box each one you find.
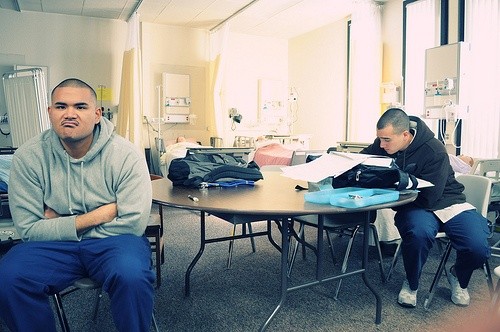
[150,175,418,332]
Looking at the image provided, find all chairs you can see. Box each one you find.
[49,275,159,332]
[142,214,165,288]
[287,210,386,300]
[205,143,295,270]
[385,173,494,308]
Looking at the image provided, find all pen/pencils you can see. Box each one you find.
[187,194,198,202]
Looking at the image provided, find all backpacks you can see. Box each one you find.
[331,163,419,191]
[167,151,264,190]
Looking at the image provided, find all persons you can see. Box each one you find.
[177,136,186,143]
[257,136,266,142]
[0,78,157,332]
[458,155,474,167]
[360,108,491,307]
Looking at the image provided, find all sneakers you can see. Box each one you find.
[397,279,419,308]
[446,269,470,306]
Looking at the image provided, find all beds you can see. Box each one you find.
[163,138,202,179]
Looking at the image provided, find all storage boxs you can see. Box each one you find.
[305,186,399,208]
